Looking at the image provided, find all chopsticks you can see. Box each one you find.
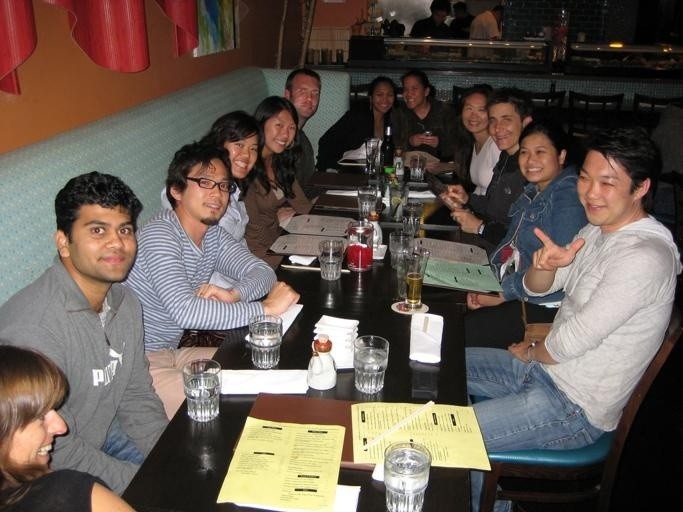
[363,398,435,451]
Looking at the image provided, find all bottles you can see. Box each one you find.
[305,47,344,66]
[305,335,336,394]
[551,6,567,63]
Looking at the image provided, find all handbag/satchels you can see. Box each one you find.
[523,323,554,346]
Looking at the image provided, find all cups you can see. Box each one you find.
[575,42,584,48]
[351,335,389,396]
[317,124,429,311]
[249,315,282,369]
[181,360,223,423]
[383,442,431,512]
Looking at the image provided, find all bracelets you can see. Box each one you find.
[526,340,539,363]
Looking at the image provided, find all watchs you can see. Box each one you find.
[477,219,485,236]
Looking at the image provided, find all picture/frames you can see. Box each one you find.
[192,0,240,58]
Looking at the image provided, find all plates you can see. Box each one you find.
[523,37,546,41]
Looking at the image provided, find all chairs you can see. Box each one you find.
[521,300,559,343]
[478,310,683,512]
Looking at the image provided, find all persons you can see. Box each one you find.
[118,139,302,423]
[314,74,400,171]
[0,344,137,511]
[159,108,267,351]
[459,82,502,194]
[463,118,588,353]
[446,87,536,257]
[271,67,323,207]
[465,121,682,454]
[241,97,313,274]
[403,0,506,59]
[1,171,170,496]
[386,68,459,163]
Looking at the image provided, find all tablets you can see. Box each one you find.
[338,157,369,167]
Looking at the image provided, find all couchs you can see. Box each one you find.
[0,63,351,308]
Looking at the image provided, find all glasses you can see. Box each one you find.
[183,174,239,194]
